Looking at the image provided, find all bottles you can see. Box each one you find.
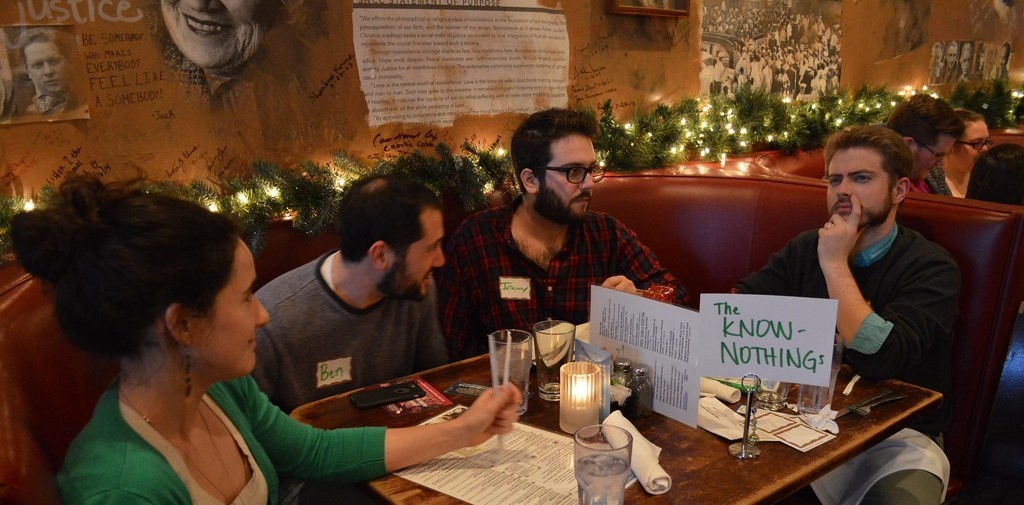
[609,357,631,386]
[627,364,653,418]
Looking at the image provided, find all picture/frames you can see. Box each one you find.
[606,0,691,16]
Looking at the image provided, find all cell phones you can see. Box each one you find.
[347,382,426,411]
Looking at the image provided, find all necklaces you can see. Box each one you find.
[118,384,244,504]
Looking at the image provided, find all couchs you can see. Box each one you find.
[0,128,1024,504]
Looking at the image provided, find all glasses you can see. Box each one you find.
[957,140,993,150]
[914,137,950,160]
[531,161,606,183]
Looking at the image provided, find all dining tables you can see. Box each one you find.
[287,297,943,505]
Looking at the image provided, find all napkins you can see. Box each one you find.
[701,376,741,403]
[600,410,672,495]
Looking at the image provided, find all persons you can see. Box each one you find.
[11,161,523,505]
[932,40,1012,86]
[735,124,962,505]
[249,175,448,418]
[11,28,80,115]
[886,93,966,194]
[923,108,989,199]
[965,143,1024,206]
[434,108,691,362]
[143,0,283,115]
[702,1,842,98]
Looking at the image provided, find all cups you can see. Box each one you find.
[489,329,533,415]
[797,334,845,414]
[753,379,790,409]
[573,424,632,505]
[533,321,576,401]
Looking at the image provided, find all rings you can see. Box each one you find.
[827,223,834,229]
